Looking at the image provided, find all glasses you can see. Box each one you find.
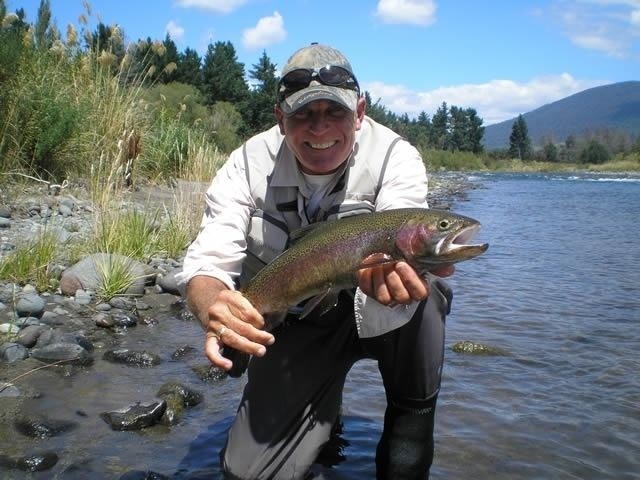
[278,64,360,98]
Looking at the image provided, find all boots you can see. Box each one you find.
[373,388,440,480]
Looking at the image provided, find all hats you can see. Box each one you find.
[278,42,360,119]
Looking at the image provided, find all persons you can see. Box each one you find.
[180,40,456,479]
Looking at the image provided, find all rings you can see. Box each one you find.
[216,328,226,339]
[204,334,220,344]
